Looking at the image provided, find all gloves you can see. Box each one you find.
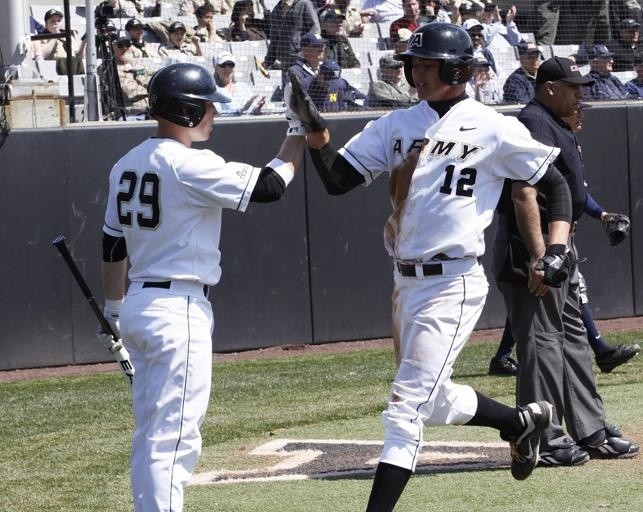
[288,68,327,135]
[95,306,122,353]
[534,244,575,288]
[283,81,307,138]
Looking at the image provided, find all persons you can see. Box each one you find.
[504,41,545,102]
[33,9,85,74]
[487,55,639,470]
[579,1,643,100]
[95,63,315,510]
[289,22,576,511]
[487,103,639,376]
[535,0,561,45]
[98,1,320,118]
[290,1,519,112]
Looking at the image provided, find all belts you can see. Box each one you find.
[396,260,442,277]
[142,281,209,297]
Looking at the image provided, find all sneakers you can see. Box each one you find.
[536,445,589,467]
[587,434,639,459]
[488,357,518,375]
[499,400,552,480]
[595,342,640,372]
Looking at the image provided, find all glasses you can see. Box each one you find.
[470,33,483,37]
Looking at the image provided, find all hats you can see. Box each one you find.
[516,41,542,54]
[393,28,413,44]
[196,4,220,16]
[105,19,185,44]
[535,56,595,86]
[618,19,640,29]
[631,52,643,66]
[325,8,346,19]
[44,9,63,20]
[458,0,496,66]
[300,32,327,45]
[588,44,615,58]
[379,52,403,68]
[213,51,235,66]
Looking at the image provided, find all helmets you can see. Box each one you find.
[145,63,231,128]
[393,24,475,85]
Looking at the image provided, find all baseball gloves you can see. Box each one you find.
[603,212,629,246]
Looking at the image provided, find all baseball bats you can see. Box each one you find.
[51,233,134,381]
